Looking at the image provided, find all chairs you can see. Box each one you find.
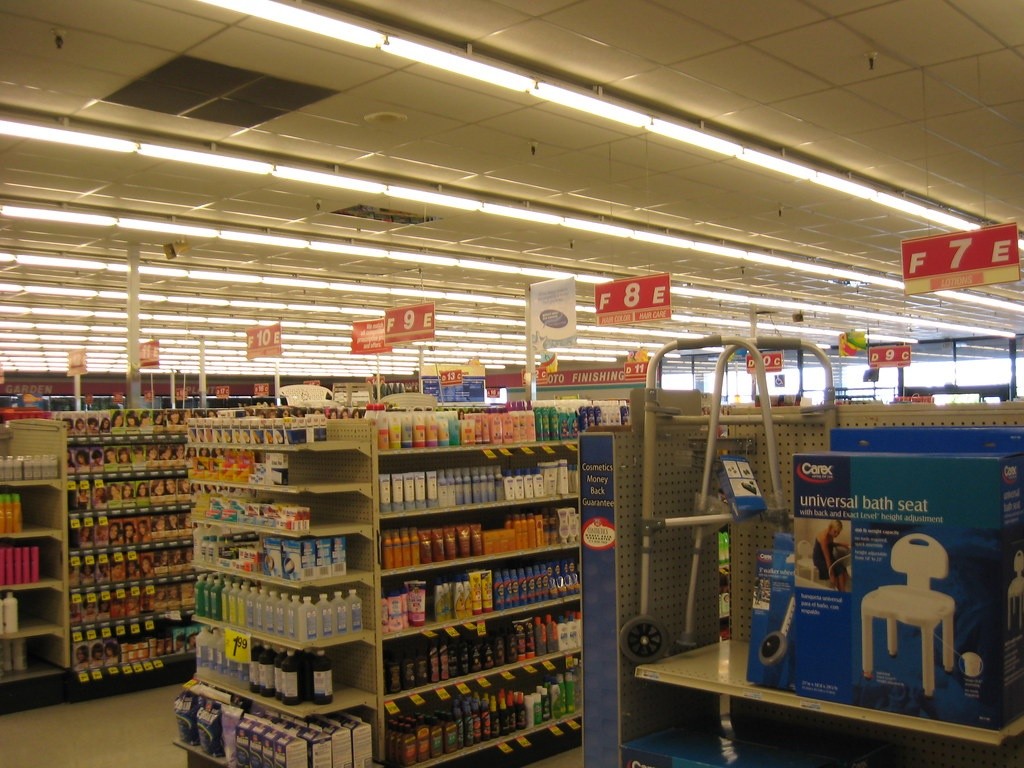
[861,532,957,699]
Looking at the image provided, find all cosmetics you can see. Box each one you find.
[192,407,628,571]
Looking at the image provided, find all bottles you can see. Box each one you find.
[387,666,576,768]
[192,522,363,706]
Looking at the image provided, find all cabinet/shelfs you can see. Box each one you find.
[1,398,1024,768]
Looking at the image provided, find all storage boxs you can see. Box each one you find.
[791,426,1024,731]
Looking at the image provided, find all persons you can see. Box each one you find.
[812,520,850,592]
[66,444,255,466]
[70,625,198,670]
[77,479,257,506]
[72,552,193,580]
[77,513,192,546]
[59,409,370,436]
[72,584,197,622]
